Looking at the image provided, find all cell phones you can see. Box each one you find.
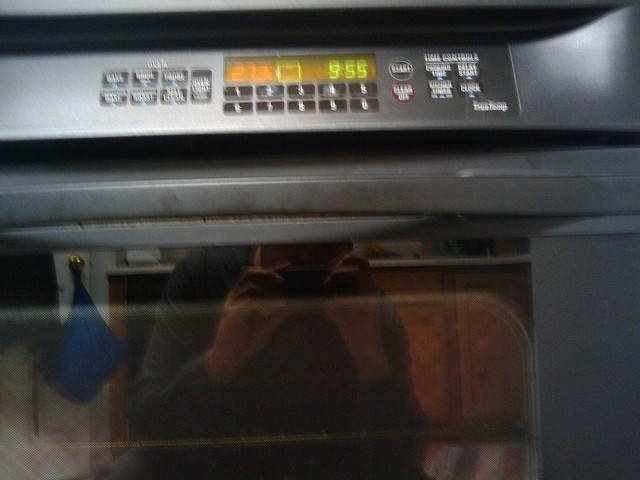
[278,272,354,296]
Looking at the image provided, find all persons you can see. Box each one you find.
[107,240,432,480]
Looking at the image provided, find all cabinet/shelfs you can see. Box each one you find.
[377,269,533,430]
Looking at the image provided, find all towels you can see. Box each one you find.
[35,272,129,405]
